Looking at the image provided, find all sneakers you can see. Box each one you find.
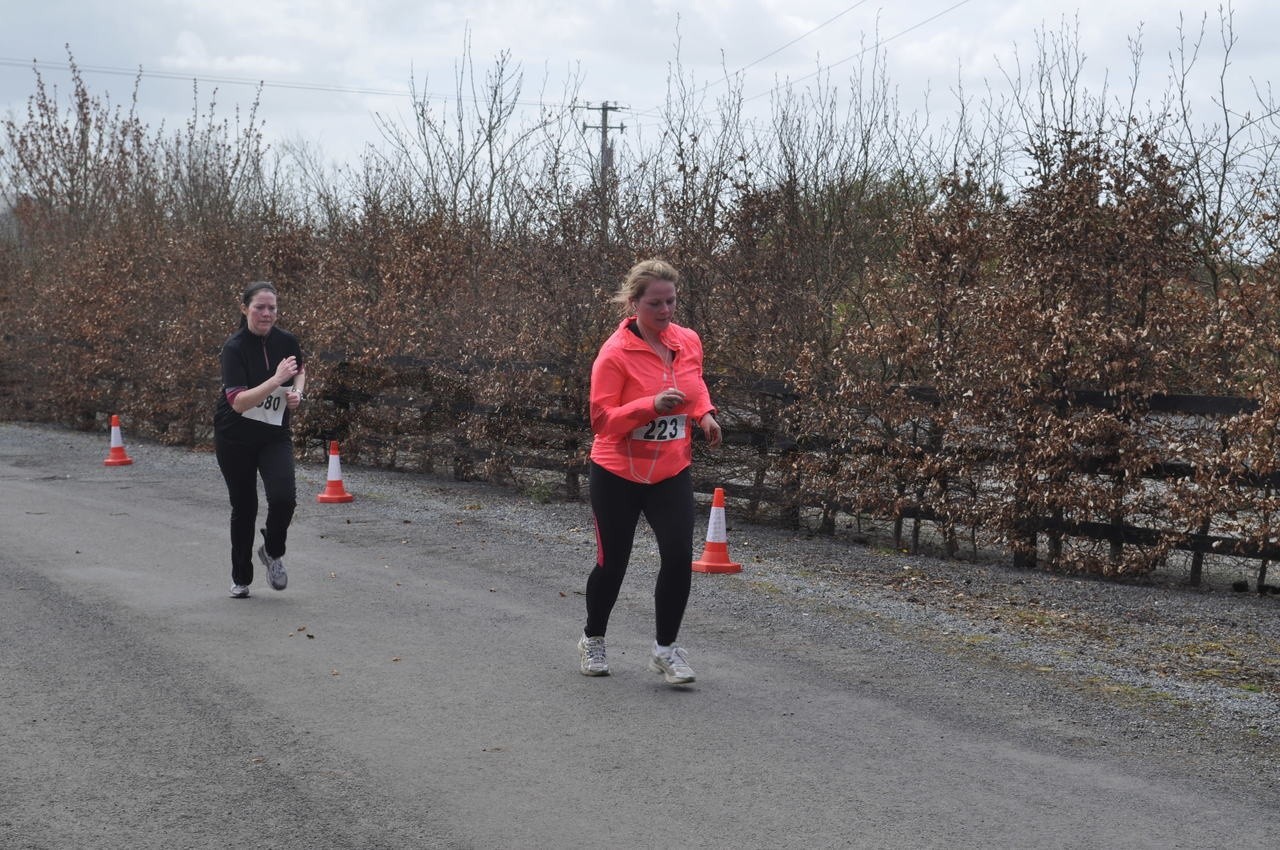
[577,632,610,676]
[229,580,249,597]
[257,543,287,590]
[650,639,696,684]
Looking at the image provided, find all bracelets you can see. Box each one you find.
[292,389,303,400]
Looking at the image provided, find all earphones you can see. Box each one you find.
[630,300,636,312]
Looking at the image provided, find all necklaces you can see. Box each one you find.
[636,324,670,382]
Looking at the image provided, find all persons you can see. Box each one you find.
[576,259,722,684]
[213,281,305,597]
